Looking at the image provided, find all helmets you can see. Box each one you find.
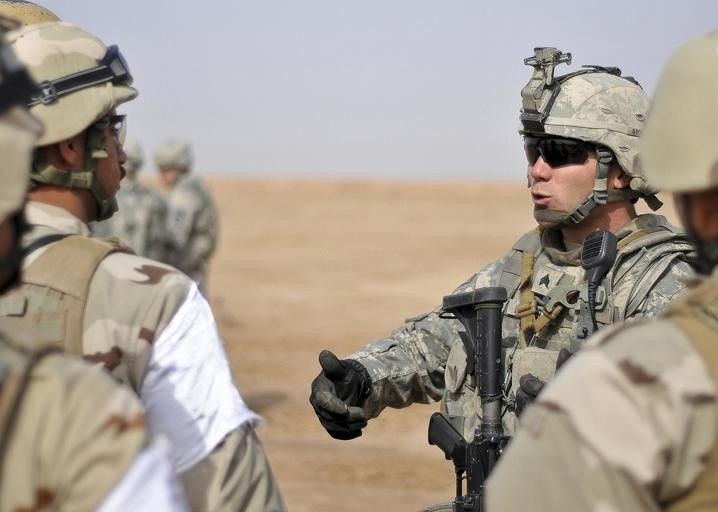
[521,32,718,195]
[1,0,138,223]
[152,135,192,171]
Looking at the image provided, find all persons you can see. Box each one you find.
[476,29,717,512]
[306,44,701,512]
[2,1,287,511]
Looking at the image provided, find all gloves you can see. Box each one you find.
[515,349,570,417]
[309,350,372,440]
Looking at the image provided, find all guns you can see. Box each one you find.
[430,288,511,512]
